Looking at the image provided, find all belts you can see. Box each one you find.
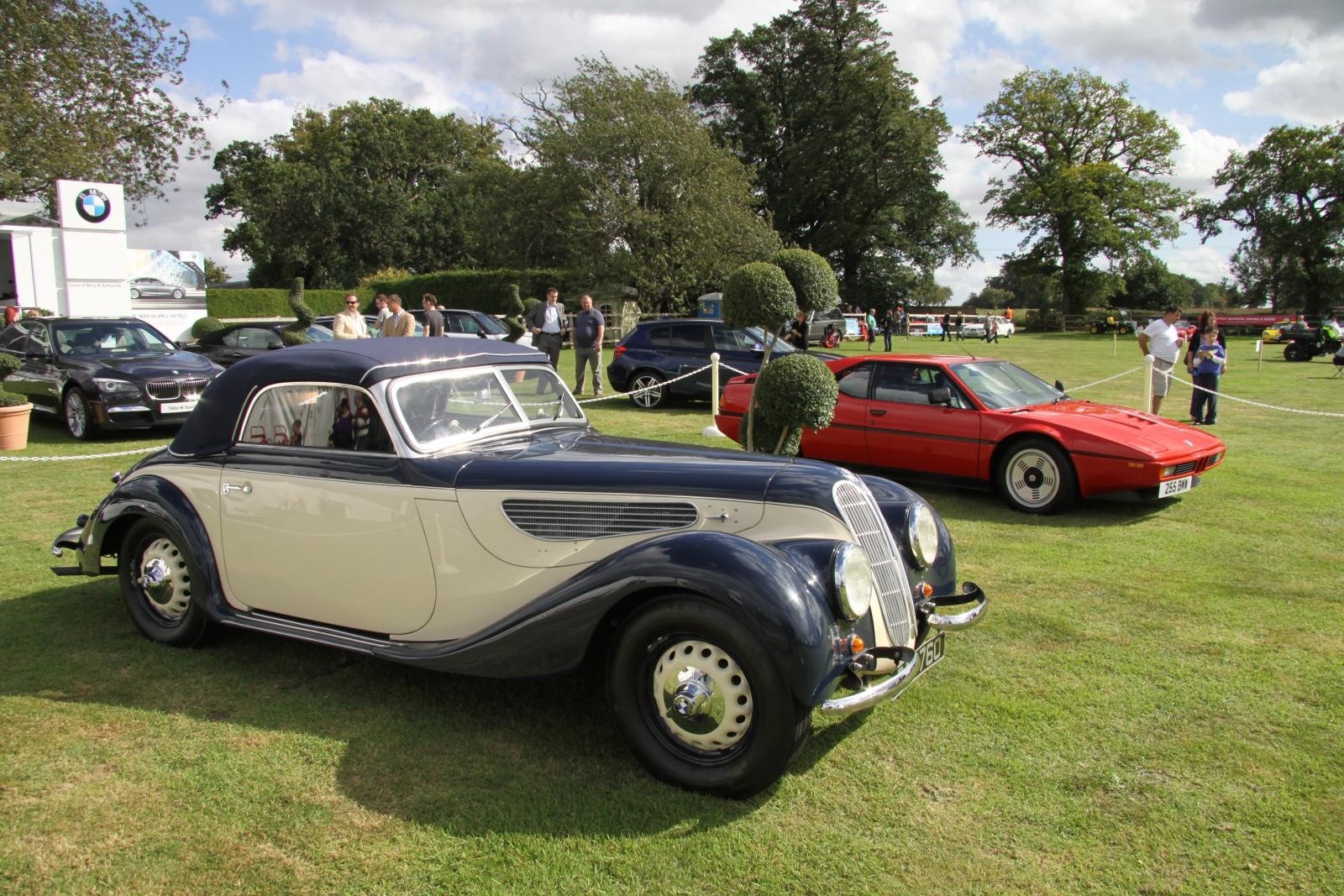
[541,332,560,336]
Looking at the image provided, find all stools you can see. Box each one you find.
[1333,364,1344,377]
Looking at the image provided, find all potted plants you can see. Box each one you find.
[0,390,34,451]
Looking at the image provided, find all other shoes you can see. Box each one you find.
[1194,419,1203,425]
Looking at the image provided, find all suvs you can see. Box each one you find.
[607,318,871,410]
[783,308,847,345]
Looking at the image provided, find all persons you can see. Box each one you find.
[292,420,302,446]
[421,294,444,337]
[334,293,369,339]
[893,307,905,336]
[1138,304,1226,425]
[1324,313,1342,336]
[571,295,605,397]
[784,311,808,350]
[866,309,876,351]
[981,312,999,343]
[941,310,951,342]
[526,288,568,396]
[955,311,964,341]
[1290,316,1306,330]
[882,310,891,352]
[375,294,392,334]
[383,295,416,336]
[1004,307,1012,319]
[328,391,370,451]
[4,306,45,355]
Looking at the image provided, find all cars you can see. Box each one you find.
[1262,322,1310,344]
[714,354,1226,517]
[962,315,1014,338]
[184,309,533,369]
[53,337,988,802]
[128,277,185,300]
[1,315,226,442]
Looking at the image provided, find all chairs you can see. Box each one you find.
[89,326,127,350]
[44,330,74,356]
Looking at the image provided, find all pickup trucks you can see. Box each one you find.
[1278,313,1344,362]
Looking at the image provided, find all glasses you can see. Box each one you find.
[346,301,357,304]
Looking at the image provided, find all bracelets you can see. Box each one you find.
[597,344,601,346]
